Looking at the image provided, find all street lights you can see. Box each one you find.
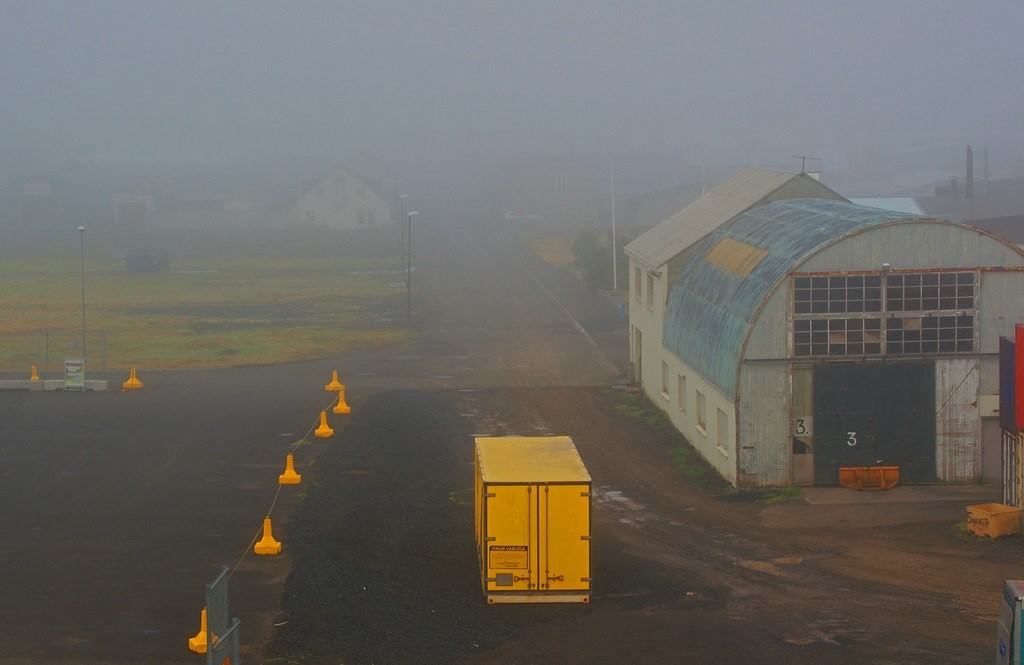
[406,210,419,328]
[77,224,85,366]
[401,193,409,278]
